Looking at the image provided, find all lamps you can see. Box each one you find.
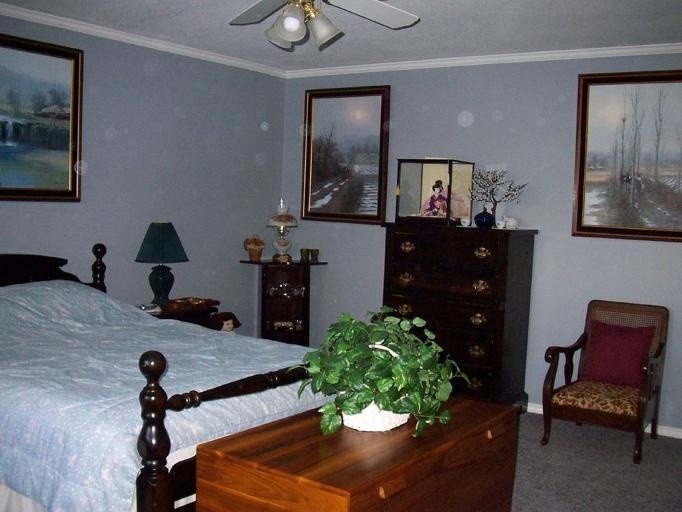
[136,220,190,304]
[264,6,345,58]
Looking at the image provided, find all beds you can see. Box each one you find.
[0,244,340,512]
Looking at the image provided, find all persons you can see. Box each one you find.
[210,312,241,333]
[429,180,446,216]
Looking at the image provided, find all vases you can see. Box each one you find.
[249,248,262,260]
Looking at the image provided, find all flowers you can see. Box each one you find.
[243,233,267,251]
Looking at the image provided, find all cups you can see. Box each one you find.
[460,219,470,227]
[299,247,321,263]
[495,220,506,229]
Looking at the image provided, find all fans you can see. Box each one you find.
[225,1,419,32]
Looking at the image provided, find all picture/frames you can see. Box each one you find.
[0,31,86,204]
[301,84,392,225]
[569,68,682,245]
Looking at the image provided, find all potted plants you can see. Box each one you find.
[286,304,470,436]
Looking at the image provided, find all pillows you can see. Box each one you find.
[577,319,656,389]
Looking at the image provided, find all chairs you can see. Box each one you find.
[540,300,670,463]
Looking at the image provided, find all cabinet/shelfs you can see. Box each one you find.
[380,221,539,411]
[258,262,311,346]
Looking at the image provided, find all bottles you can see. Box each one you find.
[473,207,494,228]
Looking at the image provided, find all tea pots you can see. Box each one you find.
[503,214,519,230]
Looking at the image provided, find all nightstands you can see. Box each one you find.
[142,297,223,325]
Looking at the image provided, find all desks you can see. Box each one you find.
[193,395,520,510]
